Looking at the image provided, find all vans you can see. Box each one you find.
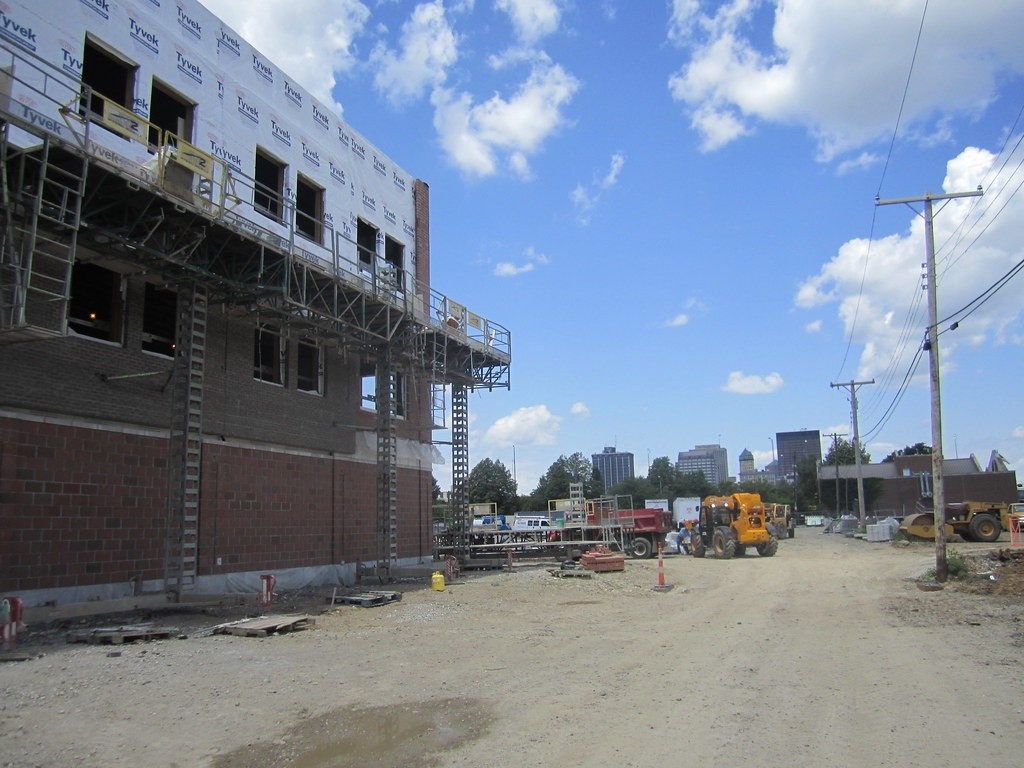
[512,517,551,528]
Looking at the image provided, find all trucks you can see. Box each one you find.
[552,506,673,562]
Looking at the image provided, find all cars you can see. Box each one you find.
[1008,502,1024,533]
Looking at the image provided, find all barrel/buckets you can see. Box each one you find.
[555,519,564,527]
[432,571,444,590]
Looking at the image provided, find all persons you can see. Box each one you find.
[551,531,561,541]
[676,528,691,553]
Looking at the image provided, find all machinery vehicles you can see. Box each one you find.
[689,492,778,559]
[897,497,1008,544]
[764,502,796,540]
